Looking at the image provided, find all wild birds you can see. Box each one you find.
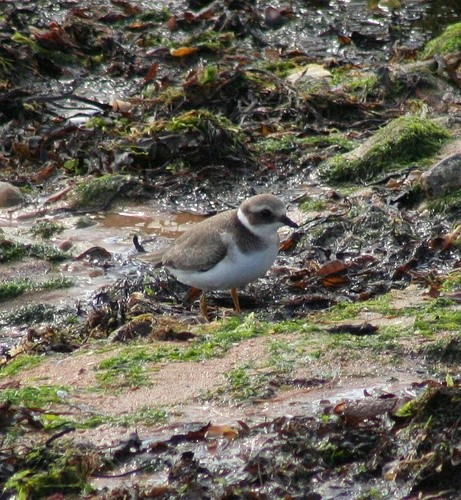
[133,194,299,320]
